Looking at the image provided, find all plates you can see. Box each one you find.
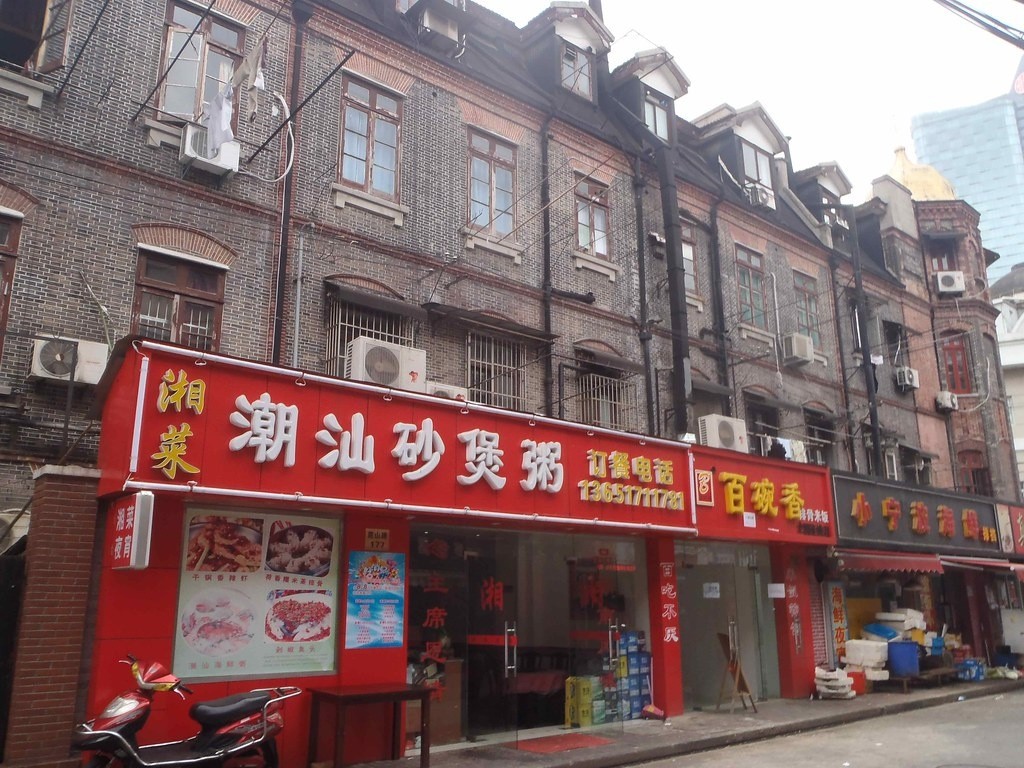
[179,587,260,658]
[265,593,332,640]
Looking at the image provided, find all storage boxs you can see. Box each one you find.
[944,634,986,682]
[565,631,651,728]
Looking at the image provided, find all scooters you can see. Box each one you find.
[71,653,302,768]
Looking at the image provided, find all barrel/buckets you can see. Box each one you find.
[848,672,866,696]
[888,641,920,676]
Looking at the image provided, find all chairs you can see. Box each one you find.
[481,651,569,730]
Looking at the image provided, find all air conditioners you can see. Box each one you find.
[698,413,748,452]
[783,333,814,363]
[27,333,109,385]
[750,184,776,211]
[418,7,459,50]
[897,366,919,389]
[343,336,467,411]
[179,121,241,178]
[933,270,965,294]
[936,392,959,410]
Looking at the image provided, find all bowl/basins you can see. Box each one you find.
[187,522,262,542]
[268,525,334,575]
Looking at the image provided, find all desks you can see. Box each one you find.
[306,684,437,768]
[505,669,568,694]
